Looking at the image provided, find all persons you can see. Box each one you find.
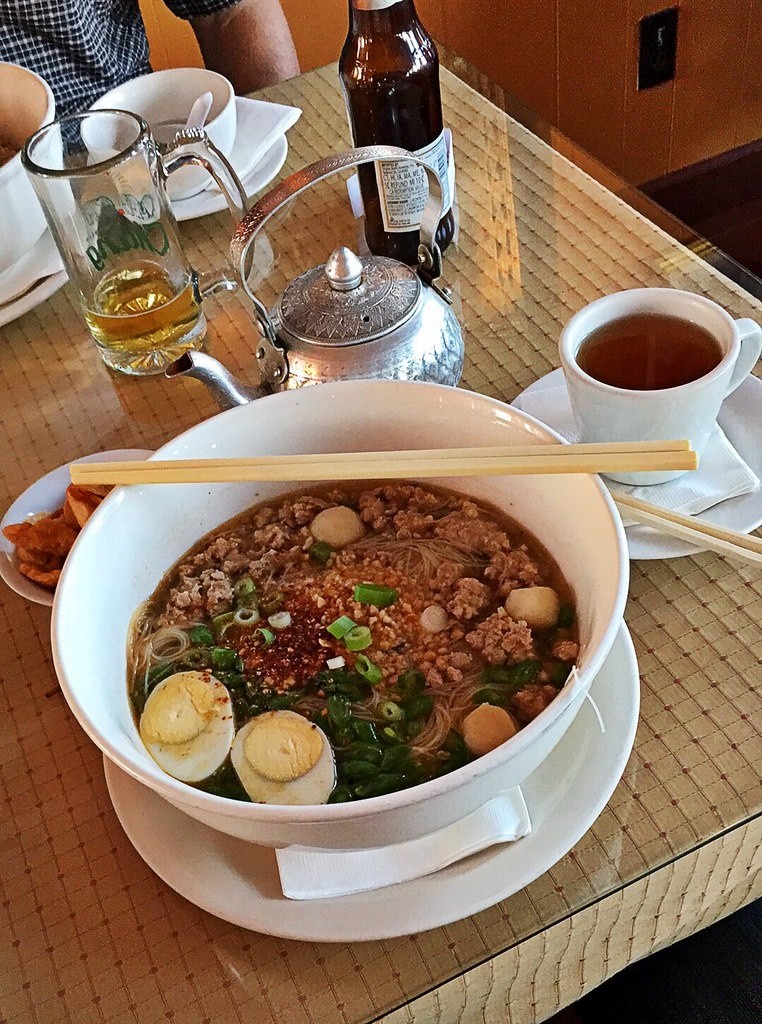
[0,0,300,158]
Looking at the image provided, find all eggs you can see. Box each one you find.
[231,709,336,806]
[140,670,236,783]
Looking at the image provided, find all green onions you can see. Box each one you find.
[138,539,575,807]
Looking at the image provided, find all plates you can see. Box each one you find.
[86,133,289,220]
[510,366,762,560]
[0,201,87,324]
[0,449,156,606]
[102,616,641,942]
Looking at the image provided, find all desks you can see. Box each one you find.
[0,38,762,1024]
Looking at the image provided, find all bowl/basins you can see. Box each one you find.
[0,60,55,273]
[50,379,630,850]
[80,68,237,201]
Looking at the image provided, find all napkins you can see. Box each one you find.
[521,383,760,529]
[0,197,88,303]
[202,95,303,194]
[275,784,532,901]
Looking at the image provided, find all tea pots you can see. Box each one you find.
[165,144,465,412]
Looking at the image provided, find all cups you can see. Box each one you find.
[558,287,762,485]
[19,109,256,377]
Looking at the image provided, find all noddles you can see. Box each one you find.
[126,480,578,755]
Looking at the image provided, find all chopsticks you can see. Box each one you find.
[611,490,762,569]
[70,438,697,486]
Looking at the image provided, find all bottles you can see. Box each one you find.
[337,0,455,266]
